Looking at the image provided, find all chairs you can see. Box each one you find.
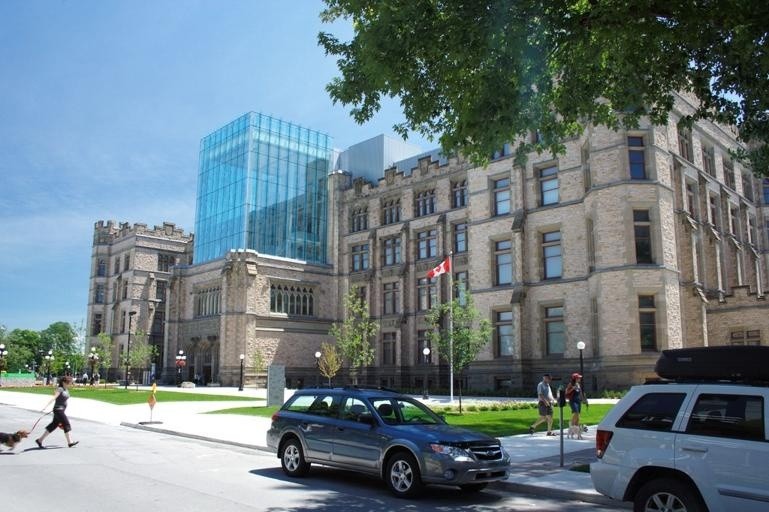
[376,404,397,425]
[682,406,765,441]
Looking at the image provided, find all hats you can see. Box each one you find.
[572,373,582,379]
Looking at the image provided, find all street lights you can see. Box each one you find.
[0,344,8,357]
[89,347,98,385]
[178,350,184,385]
[66,362,72,376]
[125,311,136,388]
[422,347,431,400]
[239,354,245,390]
[576,342,586,398]
[45,351,55,385]
[313,352,322,368]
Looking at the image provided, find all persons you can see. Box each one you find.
[93,372,101,385]
[564,372,588,435]
[82,372,88,386]
[194,372,201,384]
[529,373,557,436]
[35,375,79,449]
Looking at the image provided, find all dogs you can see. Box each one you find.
[566,421,589,441]
[0,430,30,454]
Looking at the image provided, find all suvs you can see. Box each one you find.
[266,387,510,499]
[590,346,769,512]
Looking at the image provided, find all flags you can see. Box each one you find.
[427,258,450,280]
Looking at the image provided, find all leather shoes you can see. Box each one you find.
[36,440,44,448]
[68,441,79,447]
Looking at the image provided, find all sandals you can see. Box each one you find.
[547,432,556,436]
[530,424,535,434]
